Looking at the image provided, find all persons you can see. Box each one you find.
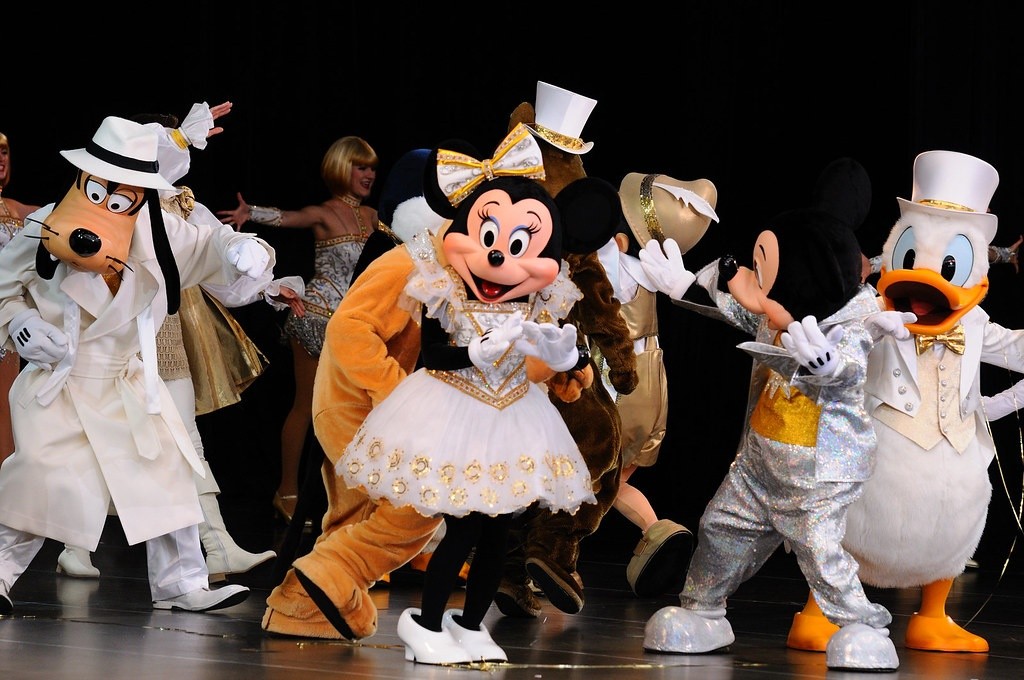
[0,134,41,464]
[57,102,306,583]
[217,136,379,527]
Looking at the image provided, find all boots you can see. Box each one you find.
[56,538,100,579]
[199,490,279,585]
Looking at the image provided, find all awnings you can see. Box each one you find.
[494,172,717,616]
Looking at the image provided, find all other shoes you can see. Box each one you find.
[273,488,313,527]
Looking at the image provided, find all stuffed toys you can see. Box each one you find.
[787,150,1024,652]
[261,241,443,642]
[335,123,597,664]
[639,157,900,672]
[0,116,251,617]
